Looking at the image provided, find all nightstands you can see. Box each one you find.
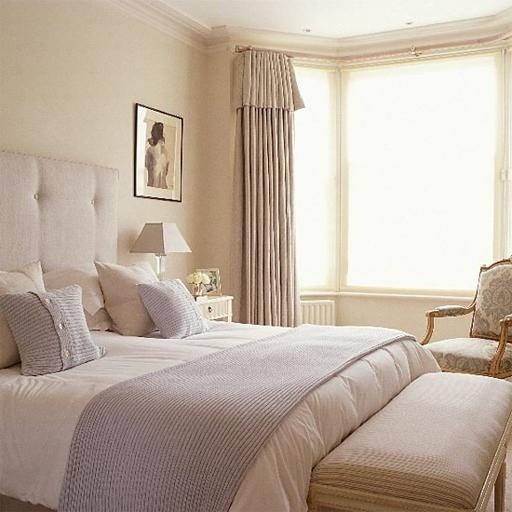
[194,296,234,322]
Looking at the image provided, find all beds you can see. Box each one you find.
[1,152,415,511]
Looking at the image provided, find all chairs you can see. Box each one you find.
[415,257,512,384]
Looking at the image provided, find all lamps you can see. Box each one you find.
[130,222,193,282]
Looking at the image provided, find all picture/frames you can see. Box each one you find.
[134,103,184,204]
[190,267,223,298]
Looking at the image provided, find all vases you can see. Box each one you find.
[187,283,203,299]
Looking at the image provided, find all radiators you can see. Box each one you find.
[299,299,336,329]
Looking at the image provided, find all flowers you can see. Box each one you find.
[183,271,210,286]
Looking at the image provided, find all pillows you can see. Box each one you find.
[136,278,210,339]
[44,260,121,331]
[94,262,160,338]
[4,284,106,377]
[0,259,47,369]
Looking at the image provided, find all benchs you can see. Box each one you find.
[306,373,512,512]
